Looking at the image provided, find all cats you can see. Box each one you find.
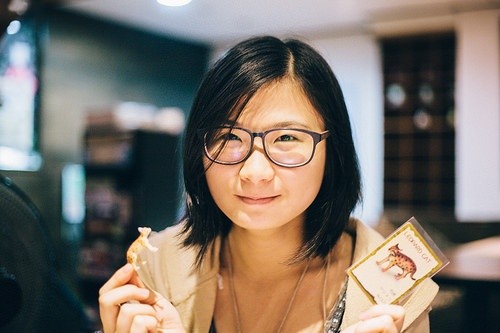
[127,227,159,271]
[376,243,417,280]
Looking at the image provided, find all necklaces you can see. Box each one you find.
[226,238,316,333]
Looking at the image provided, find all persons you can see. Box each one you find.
[97,35,441,333]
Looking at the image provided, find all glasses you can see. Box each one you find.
[203,126,329,168]
